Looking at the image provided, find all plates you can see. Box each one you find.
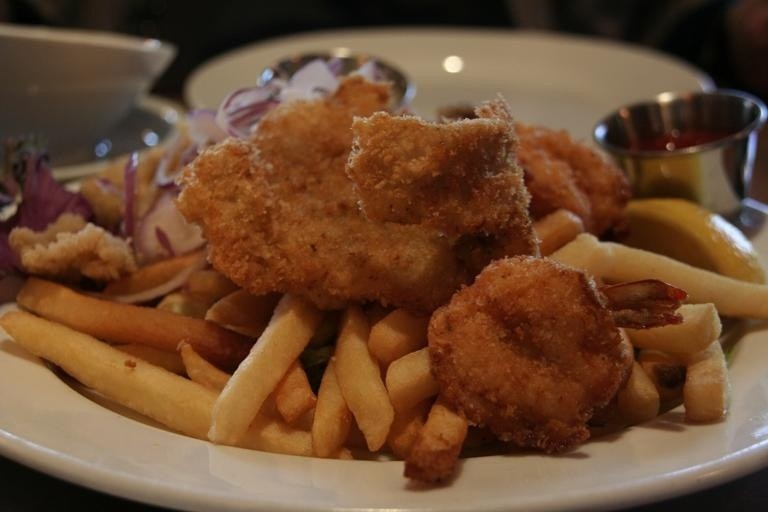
[5,23,767,512]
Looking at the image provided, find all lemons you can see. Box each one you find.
[624,198,768,284]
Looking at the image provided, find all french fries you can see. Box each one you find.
[0,233,768,487]
